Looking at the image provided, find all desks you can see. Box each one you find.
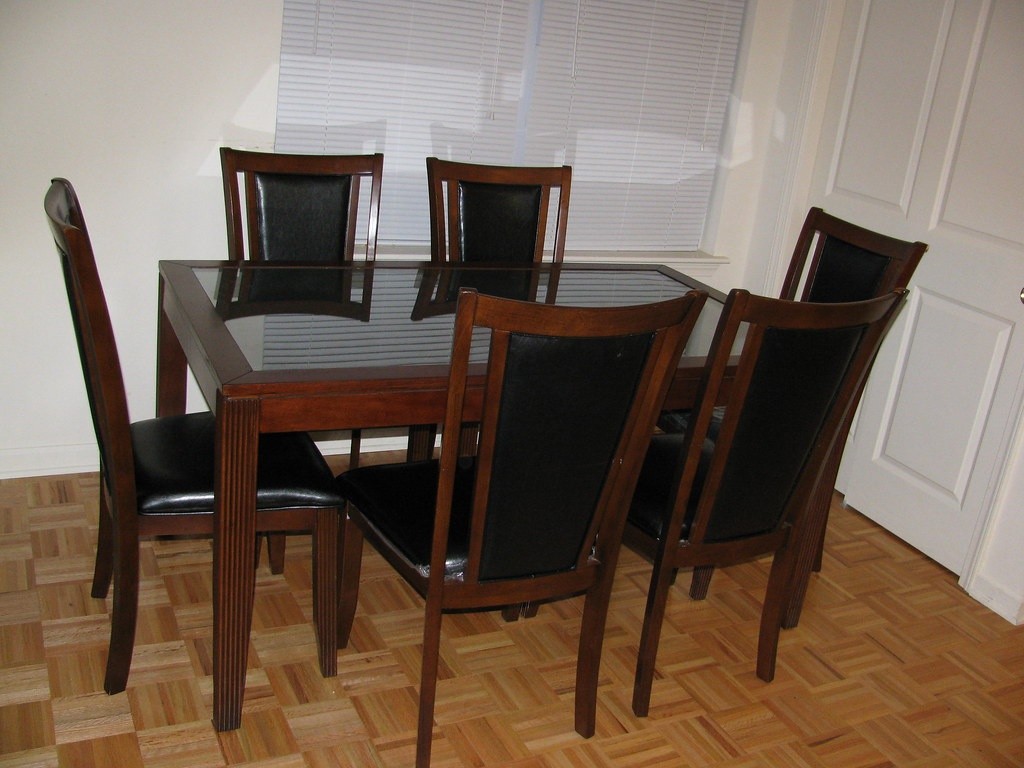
[158,260,820,732]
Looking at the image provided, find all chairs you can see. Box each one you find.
[45,178,347,695]
[524,288,907,719]
[684,208,926,600]
[218,146,384,574]
[410,156,571,463]
[329,286,710,768]
